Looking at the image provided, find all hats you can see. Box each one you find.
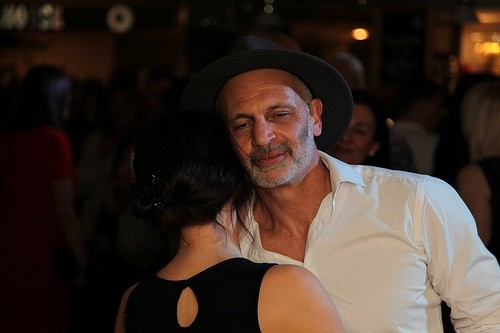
[187,36,354,153]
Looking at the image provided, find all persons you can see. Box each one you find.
[114,111,346,333]
[167,33,500,333]
[303,35,500,266]
[0,62,248,333]
